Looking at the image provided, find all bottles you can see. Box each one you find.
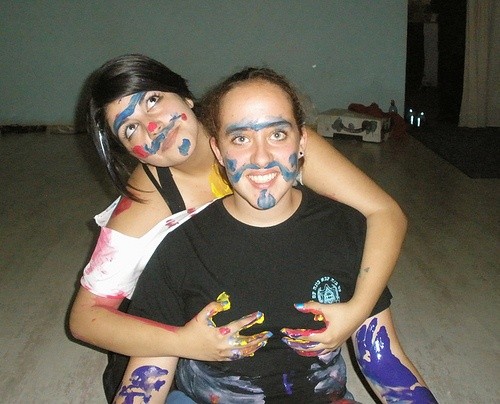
[406,109,416,128]
[417,111,426,128]
[389,100,397,113]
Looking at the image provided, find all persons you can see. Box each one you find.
[68,55,408,404]
[114,69,437,404]
